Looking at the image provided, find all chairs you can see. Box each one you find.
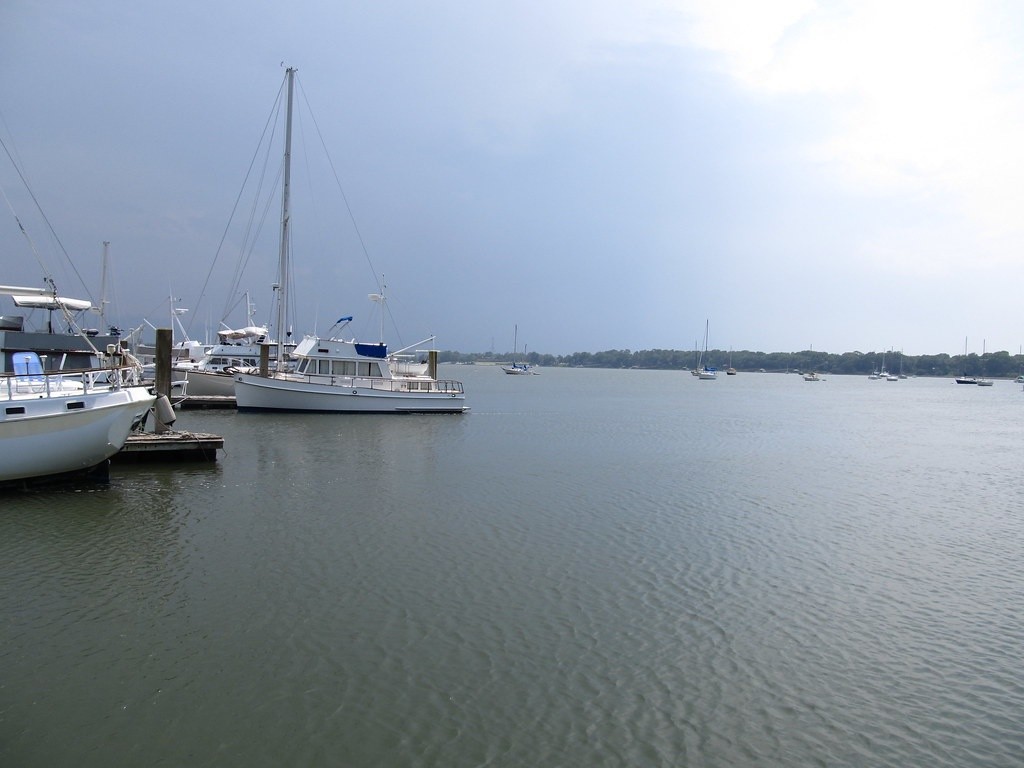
[12,352,65,394]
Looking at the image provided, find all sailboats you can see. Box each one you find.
[691,318,738,380]
[956,337,978,384]
[867,345,908,382]
[1014,345,1024,384]
[501,324,534,375]
[978,338,995,387]
[1,67,473,490]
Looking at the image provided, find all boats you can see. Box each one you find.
[804,376,820,381]
